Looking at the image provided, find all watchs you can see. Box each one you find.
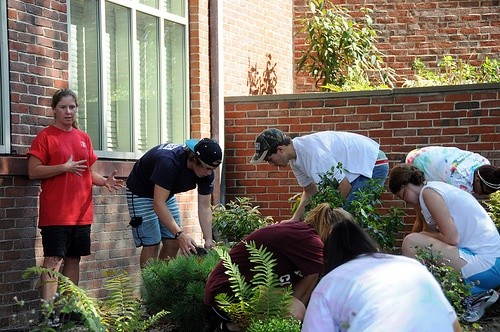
[174,231,183,238]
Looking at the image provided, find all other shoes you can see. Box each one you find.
[37,312,62,328]
[60,310,87,326]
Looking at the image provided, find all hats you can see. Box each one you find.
[250,128,286,164]
[185,138,222,169]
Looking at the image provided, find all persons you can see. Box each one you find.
[301,221,463,332]
[203,203,354,332]
[401,144,500,233]
[389,163,500,323]
[250,128,389,221]
[27,88,124,326]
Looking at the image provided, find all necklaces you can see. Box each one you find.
[126,137,223,276]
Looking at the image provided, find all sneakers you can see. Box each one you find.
[461,288,499,322]
